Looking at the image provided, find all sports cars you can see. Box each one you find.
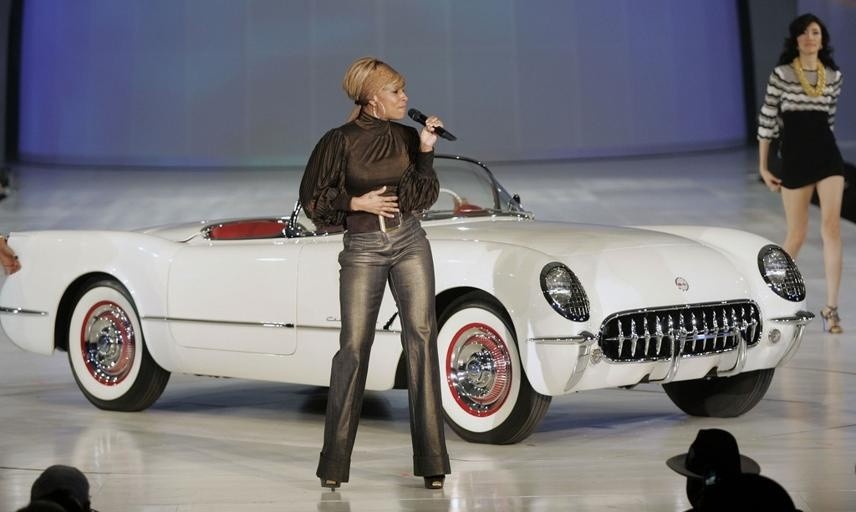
[0,151,818,446]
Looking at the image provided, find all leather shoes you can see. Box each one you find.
[30,464,91,504]
[424,475,446,490]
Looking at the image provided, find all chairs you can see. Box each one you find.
[210,218,306,242]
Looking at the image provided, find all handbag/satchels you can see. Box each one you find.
[767,139,797,179]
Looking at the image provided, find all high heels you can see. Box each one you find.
[320,478,341,492]
[819,305,842,336]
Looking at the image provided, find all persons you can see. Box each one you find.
[756,11,850,334]
[16,465,96,512]
[299,55,450,491]
[665,426,760,512]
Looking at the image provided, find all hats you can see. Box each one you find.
[666,427,761,480]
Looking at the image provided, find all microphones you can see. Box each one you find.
[408,108,457,142]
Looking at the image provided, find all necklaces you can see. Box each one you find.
[791,55,827,98]
